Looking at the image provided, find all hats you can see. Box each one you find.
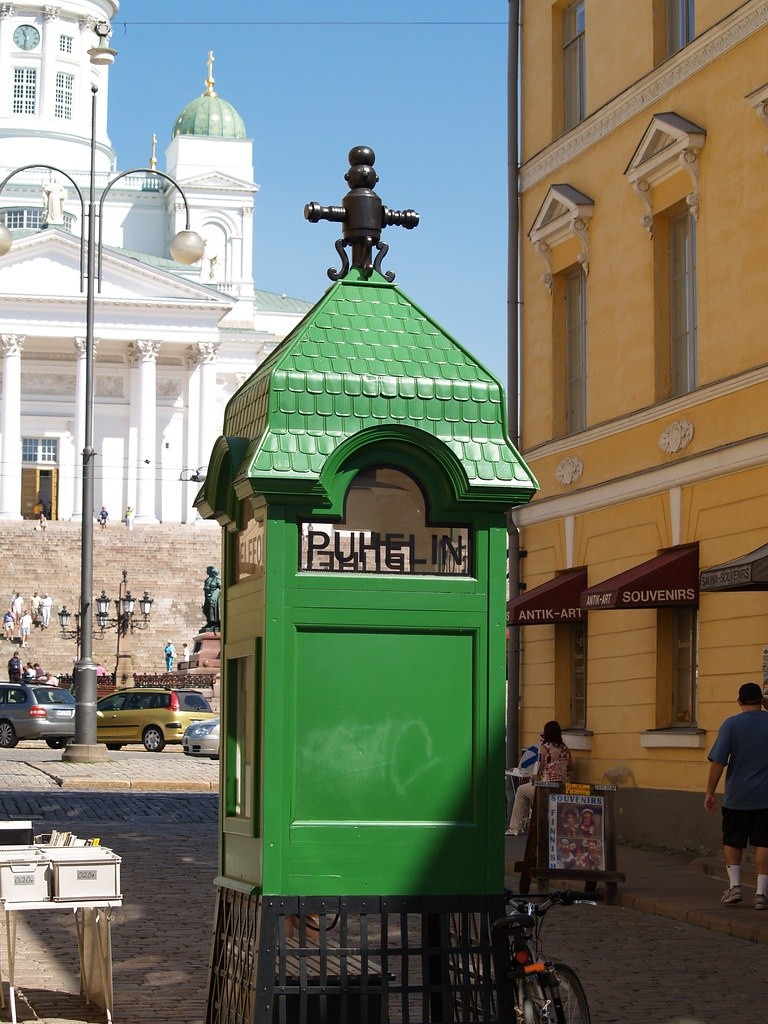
[168,640,173,644]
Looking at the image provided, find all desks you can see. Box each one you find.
[505,770,537,829]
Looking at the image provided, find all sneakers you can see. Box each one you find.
[721,885,743,904]
[752,893,768,910]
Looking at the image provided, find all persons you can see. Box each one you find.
[41,178,63,221]
[7,651,44,683]
[100,507,108,529]
[704,683,768,909]
[38,513,48,530]
[126,507,133,530]
[34,500,51,519]
[45,672,58,700]
[203,566,221,627]
[164,641,175,672]
[96,664,105,675]
[181,643,189,661]
[2,592,53,647]
[505,721,574,835]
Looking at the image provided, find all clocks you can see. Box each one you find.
[13,25,40,50]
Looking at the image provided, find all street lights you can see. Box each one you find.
[96,569,154,691]
[57,606,106,677]
[0,84,206,763]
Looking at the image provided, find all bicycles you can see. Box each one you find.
[492,887,606,1024]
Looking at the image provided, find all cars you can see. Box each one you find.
[182,717,220,760]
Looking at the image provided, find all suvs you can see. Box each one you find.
[97,687,216,752]
[0,681,76,749]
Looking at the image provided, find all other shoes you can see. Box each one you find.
[20,643,26,648]
[505,828,525,836]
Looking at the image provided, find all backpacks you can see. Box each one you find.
[11,658,19,669]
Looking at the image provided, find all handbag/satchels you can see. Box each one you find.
[102,673,105,676]
[171,652,177,658]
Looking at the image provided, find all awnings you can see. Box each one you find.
[506,570,587,626]
[700,543,768,592]
[580,546,699,610]
